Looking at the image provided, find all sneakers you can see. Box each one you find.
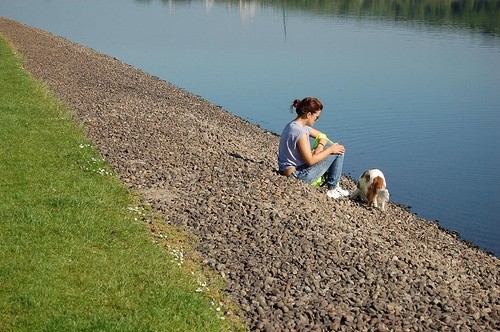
[326,187,349,199]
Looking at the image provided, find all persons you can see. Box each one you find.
[278,97,350,199]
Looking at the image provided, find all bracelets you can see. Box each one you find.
[318,143,325,147]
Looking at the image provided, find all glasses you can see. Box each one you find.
[311,113,319,122]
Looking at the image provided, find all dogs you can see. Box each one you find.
[349,168,390,212]
